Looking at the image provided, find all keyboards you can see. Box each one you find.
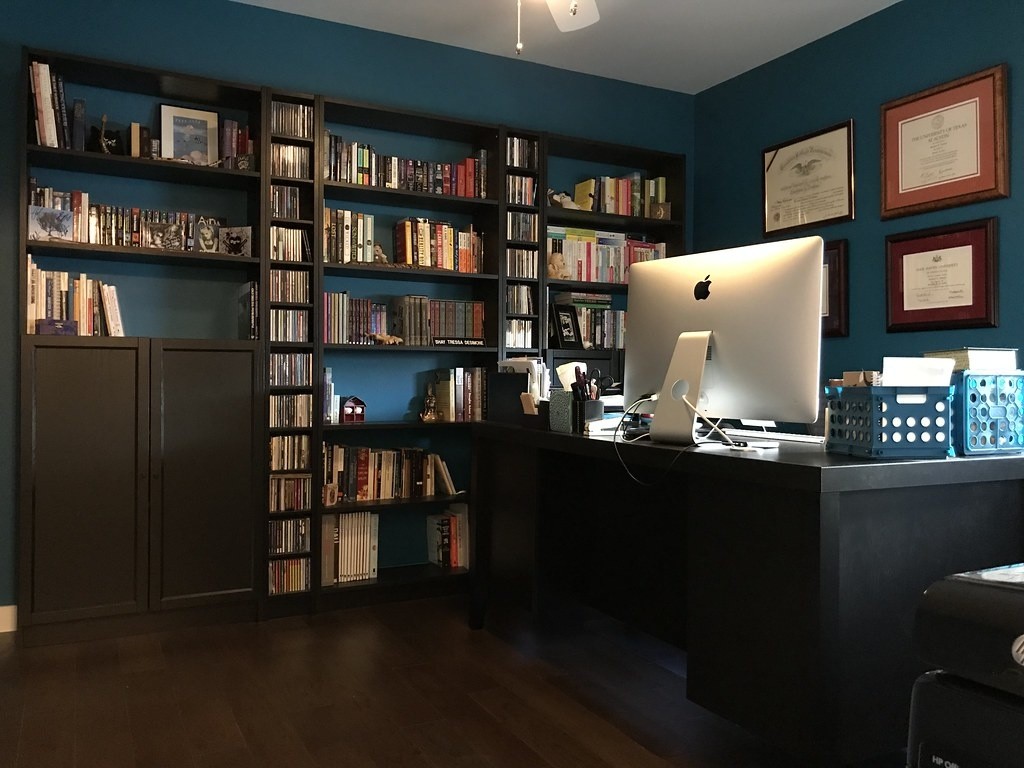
[721,427,826,444]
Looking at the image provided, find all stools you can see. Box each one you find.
[903,562,1024,768]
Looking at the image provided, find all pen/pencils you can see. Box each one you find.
[570,367,601,401]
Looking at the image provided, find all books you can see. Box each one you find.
[922,348,1018,374]
[237,281,258,339]
[435,366,486,423]
[27,177,253,257]
[504,136,538,349]
[324,288,486,347]
[830,370,882,444]
[270,99,315,595]
[321,198,484,275]
[220,120,255,171]
[428,501,469,569]
[575,171,671,219]
[546,226,665,284]
[548,293,627,350]
[26,254,124,336]
[321,439,459,506]
[28,61,86,151]
[323,366,341,425]
[127,122,160,162]
[497,356,550,403]
[322,127,488,198]
[319,510,380,587]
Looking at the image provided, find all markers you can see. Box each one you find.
[643,413,654,418]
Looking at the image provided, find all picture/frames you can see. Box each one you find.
[549,303,584,351]
[882,214,1000,332]
[879,61,1012,221]
[760,118,857,239]
[821,238,849,338]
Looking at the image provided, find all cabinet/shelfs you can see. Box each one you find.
[12,44,684,652]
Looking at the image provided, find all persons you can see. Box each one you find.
[562,313,574,339]
[420,382,444,424]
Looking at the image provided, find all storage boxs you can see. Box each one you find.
[548,388,575,433]
[950,369,1024,456]
[520,413,550,431]
[824,385,956,460]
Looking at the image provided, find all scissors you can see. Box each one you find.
[591,368,614,393]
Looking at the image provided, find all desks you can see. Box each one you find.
[468,418,1024,768]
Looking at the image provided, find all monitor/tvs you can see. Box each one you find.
[626,236,824,447]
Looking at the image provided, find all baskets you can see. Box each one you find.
[825,387,956,462]
[952,368,1023,457]
